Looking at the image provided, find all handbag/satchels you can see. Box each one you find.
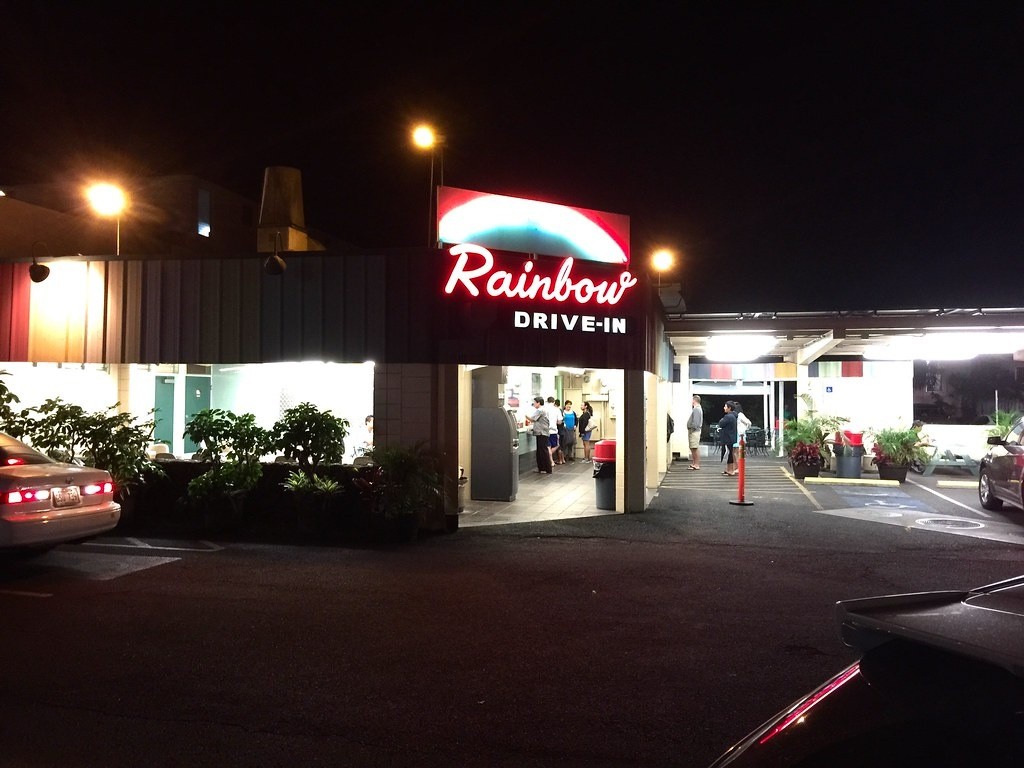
[562,426,577,448]
[584,411,597,432]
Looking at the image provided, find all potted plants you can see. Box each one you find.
[776,393,851,479]
[266,407,346,534]
[183,409,266,531]
[866,427,929,483]
[378,446,429,544]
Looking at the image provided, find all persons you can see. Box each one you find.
[719,401,751,475]
[910,421,928,472]
[579,402,593,463]
[525,396,552,473]
[687,396,703,470]
[667,413,670,443]
[360,415,373,447]
[554,399,565,464]
[563,400,578,461]
[545,397,563,467]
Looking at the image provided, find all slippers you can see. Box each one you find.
[720,469,735,476]
[687,464,700,470]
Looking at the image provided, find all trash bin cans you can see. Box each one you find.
[832,430,864,478]
[592,440,616,510]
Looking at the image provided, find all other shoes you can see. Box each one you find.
[541,471,547,473]
[579,458,586,464]
[533,469,539,472]
[734,468,738,473]
[585,459,591,463]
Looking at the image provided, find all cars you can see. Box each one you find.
[978,416,1024,510]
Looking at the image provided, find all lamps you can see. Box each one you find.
[264,232,287,273]
[29,240,52,283]
